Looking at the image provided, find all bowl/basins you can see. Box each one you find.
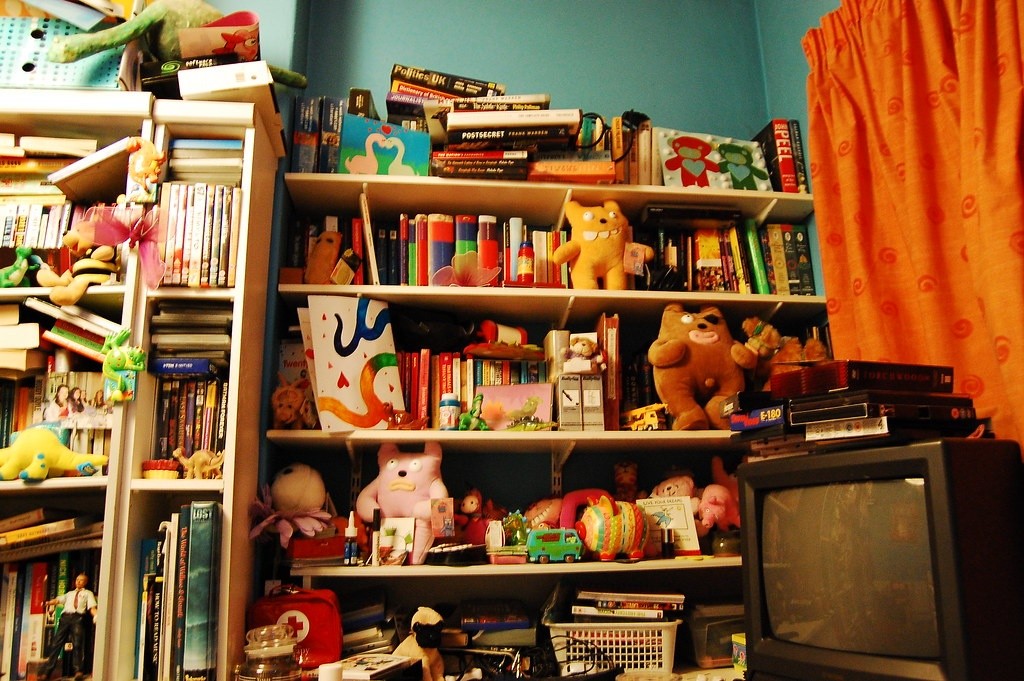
[712,530,741,557]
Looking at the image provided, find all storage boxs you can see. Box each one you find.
[563,359,597,375]
[282,515,349,567]
[728,403,789,430]
[569,332,598,353]
[552,373,583,432]
[769,358,955,398]
[179,59,289,160]
[688,616,745,668]
[581,373,605,431]
[544,582,683,677]
[719,391,774,419]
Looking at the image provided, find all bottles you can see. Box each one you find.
[439,393,461,430]
[344,511,358,566]
[518,242,534,283]
[239,625,302,681]
[319,664,342,681]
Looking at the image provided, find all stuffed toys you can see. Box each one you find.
[713,457,739,503]
[36,219,119,306]
[561,337,603,375]
[649,304,756,432]
[553,197,654,291]
[762,337,803,391]
[802,332,826,361]
[454,490,482,525]
[356,442,447,565]
[699,484,741,533]
[0,428,109,481]
[248,462,331,548]
[652,476,708,537]
[742,317,781,357]
[392,607,445,681]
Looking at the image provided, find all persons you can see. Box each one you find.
[91,390,106,408]
[70,388,84,413]
[43,384,70,420]
[79,390,89,407]
[36,574,97,681]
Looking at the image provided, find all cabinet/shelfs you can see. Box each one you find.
[0,83,829,681]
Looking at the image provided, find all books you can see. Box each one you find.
[273,296,837,431]
[151,298,232,476]
[305,579,537,681]
[278,196,816,298]
[572,585,687,623]
[137,501,220,681]
[0,507,103,681]
[0,132,241,294]
[289,62,808,195]
[0,298,123,479]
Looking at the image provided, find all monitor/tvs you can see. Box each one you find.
[739,438,1024,681]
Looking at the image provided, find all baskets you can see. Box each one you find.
[543,582,682,672]
